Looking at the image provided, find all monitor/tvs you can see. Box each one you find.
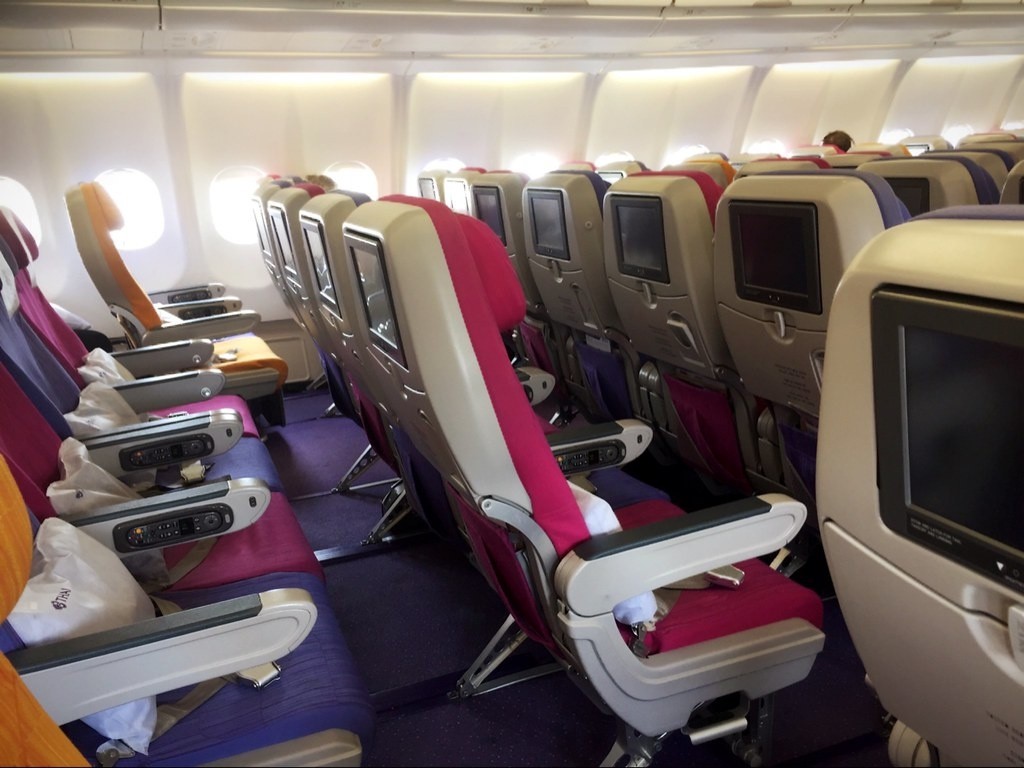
[886,177,931,217]
[255,202,410,378]
[418,172,824,317]
[907,145,930,157]
[871,291,1024,595]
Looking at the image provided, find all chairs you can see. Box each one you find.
[1,124,1024,768]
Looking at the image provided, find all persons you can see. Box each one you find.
[822,128,854,151]
